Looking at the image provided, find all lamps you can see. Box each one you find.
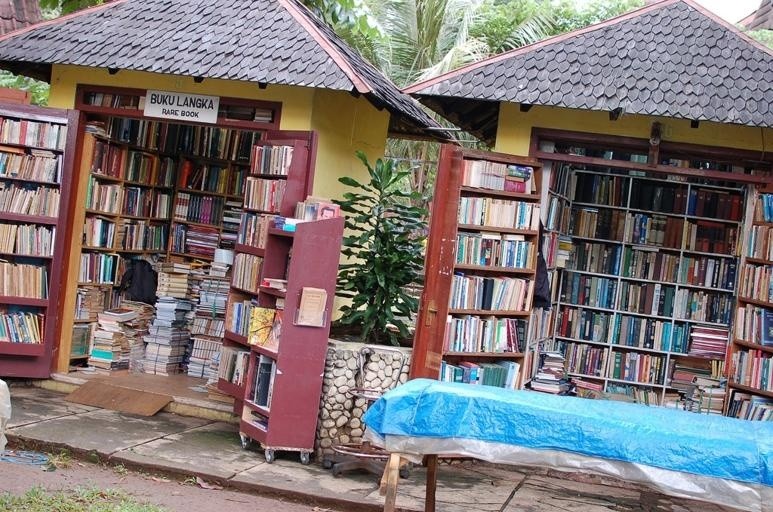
[648,120,662,145]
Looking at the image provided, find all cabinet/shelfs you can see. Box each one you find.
[74,91,265,393]
[549,159,734,414]
[221,132,336,450]
[410,143,550,385]
[731,186,772,419]
[1,100,74,386]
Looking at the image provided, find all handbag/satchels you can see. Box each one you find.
[532,250,549,311]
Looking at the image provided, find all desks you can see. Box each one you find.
[355,375,773,511]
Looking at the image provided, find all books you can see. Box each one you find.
[454,159,541,269]
[0,117,68,220]
[85,89,273,163]
[522,339,727,416]
[540,232,735,360]
[1,223,57,344]
[69,251,285,434]
[720,263,771,423]
[78,215,166,285]
[167,159,243,259]
[232,144,339,293]
[441,275,535,390]
[539,141,771,255]
[85,142,172,220]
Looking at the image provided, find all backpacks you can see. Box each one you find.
[116,260,158,303]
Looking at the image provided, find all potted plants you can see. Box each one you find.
[310,150,429,467]
